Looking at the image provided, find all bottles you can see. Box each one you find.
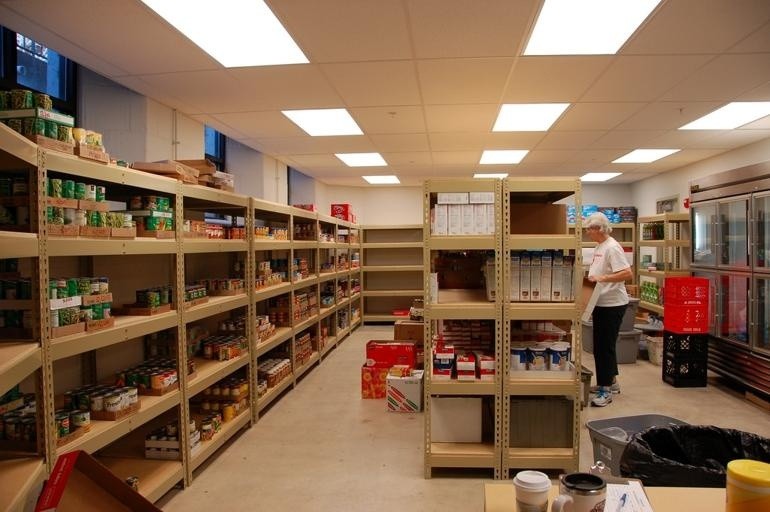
[643,223,663,240]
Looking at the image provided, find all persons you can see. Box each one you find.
[585,212,633,407]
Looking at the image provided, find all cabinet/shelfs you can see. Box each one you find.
[689,160,770,395]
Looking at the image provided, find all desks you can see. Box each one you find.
[484,482,728,511]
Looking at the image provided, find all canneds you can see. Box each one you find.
[145,325,210,359]
[145,419,200,452]
[257,357,291,388]
[197,375,268,422]
[1,276,112,337]
[255,226,274,241]
[1,176,137,240]
[232,261,286,285]
[1,385,90,448]
[270,311,284,323]
[116,357,196,388]
[512,340,571,370]
[63,382,139,412]
[184,219,226,239]
[200,414,222,441]
[136,284,207,307]
[271,228,288,240]
[190,279,264,294]
[200,334,249,360]
[217,314,276,338]
[227,227,247,239]
[129,194,176,232]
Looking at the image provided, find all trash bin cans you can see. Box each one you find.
[585,414,770,487]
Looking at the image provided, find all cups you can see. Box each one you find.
[512,470,551,512]
[724,457,770,511]
[550,471,607,512]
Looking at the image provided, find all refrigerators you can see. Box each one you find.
[691,195,770,354]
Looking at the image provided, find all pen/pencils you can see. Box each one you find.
[615,492,627,512]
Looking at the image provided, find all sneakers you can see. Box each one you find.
[590,386,613,408]
[589,379,621,395]
